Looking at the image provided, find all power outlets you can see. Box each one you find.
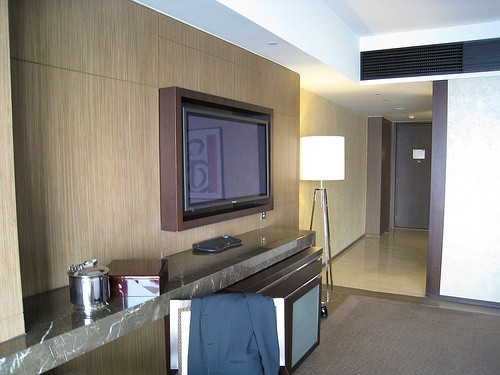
[260,235,267,246]
[261,211,266,220]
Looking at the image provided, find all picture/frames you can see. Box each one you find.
[187,127,225,200]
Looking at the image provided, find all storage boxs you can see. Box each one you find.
[106,258,169,297]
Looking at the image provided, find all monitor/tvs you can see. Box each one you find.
[182,106,269,212]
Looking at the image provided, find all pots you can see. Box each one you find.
[67,260,110,306]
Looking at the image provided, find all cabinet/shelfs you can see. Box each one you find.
[164,246,323,375]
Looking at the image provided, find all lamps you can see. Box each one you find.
[299,135,346,305]
